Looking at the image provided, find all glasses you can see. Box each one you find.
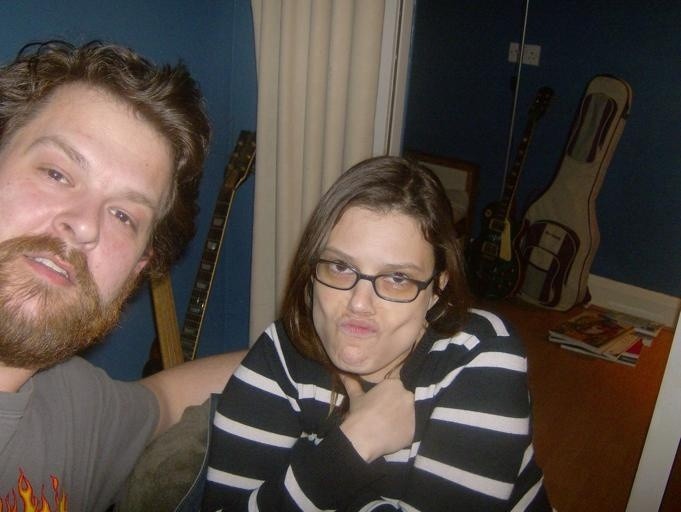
[315,258,438,302]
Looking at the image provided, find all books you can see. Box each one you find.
[547,304,662,368]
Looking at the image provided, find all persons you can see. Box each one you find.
[200,156,556,511]
[0,37,257,511]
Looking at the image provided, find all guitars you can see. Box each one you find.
[141,130,257,377]
[465,86,555,302]
